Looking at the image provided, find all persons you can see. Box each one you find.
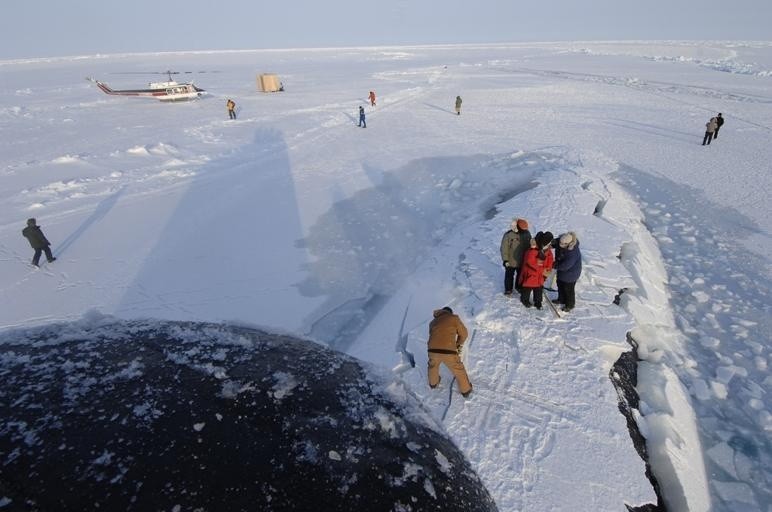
[227,99,236,120]
[500,217,532,298]
[358,106,366,128]
[455,96,462,115]
[427,306,472,398]
[21,219,57,269]
[701,117,718,146]
[714,113,724,139]
[368,91,376,106]
[550,231,582,312]
[518,231,554,310]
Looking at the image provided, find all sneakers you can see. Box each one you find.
[503,289,514,297]
[551,298,565,305]
[515,288,522,294]
[562,304,576,312]
[428,375,442,390]
[30,262,40,269]
[462,382,473,398]
[534,303,543,310]
[521,298,532,309]
[48,256,57,264]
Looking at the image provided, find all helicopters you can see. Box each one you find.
[83,70,222,103]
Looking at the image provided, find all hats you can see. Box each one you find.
[518,220,573,247]
